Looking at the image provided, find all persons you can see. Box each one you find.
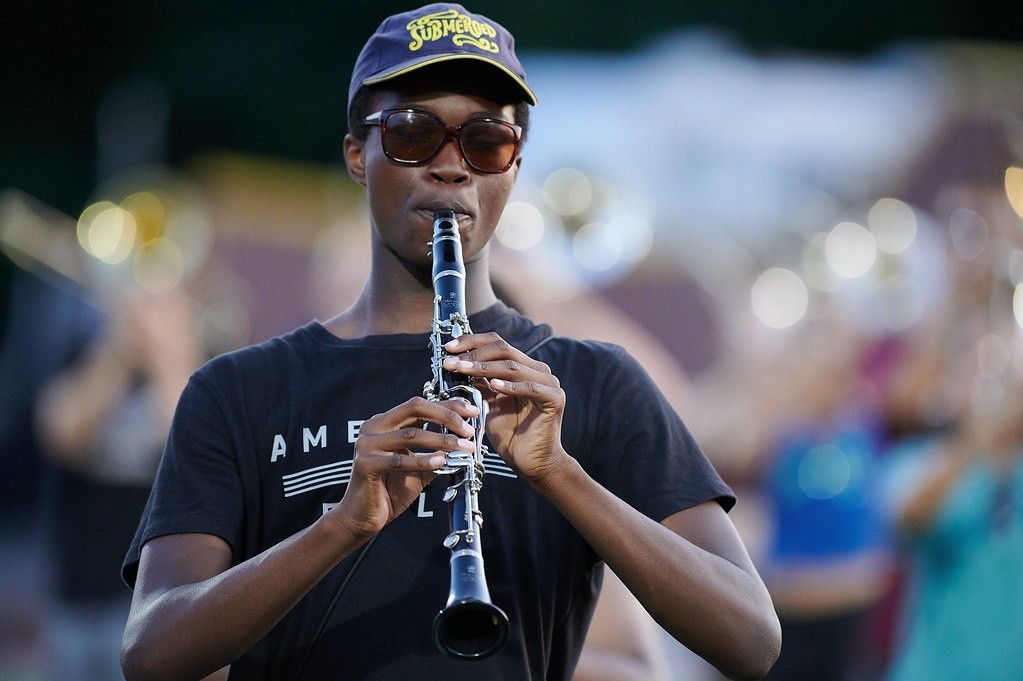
[574,182,1023,680]
[119,3,783,681]
[0,214,370,681]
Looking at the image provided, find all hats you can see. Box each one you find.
[346,2,539,131]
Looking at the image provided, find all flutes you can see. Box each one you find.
[422,205,513,663]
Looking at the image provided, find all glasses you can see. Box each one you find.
[363,107,523,175]
